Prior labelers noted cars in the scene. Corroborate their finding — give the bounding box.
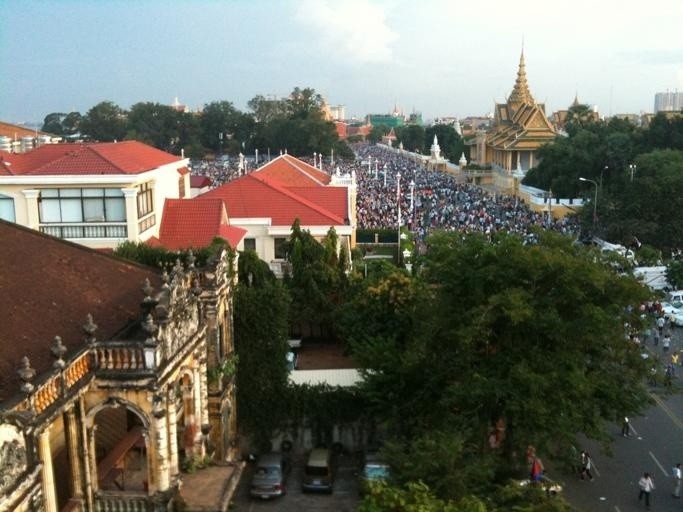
[660,290,683,327]
[354,455,394,495]
[249,453,292,501]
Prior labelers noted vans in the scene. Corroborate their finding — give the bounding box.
[302,447,337,494]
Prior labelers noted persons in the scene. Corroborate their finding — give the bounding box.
[191,156,268,189]
[671,463,682,498]
[637,472,655,506]
[623,299,680,387]
[297,141,578,244]
[621,417,633,438]
[580,454,594,482]
[580,450,585,465]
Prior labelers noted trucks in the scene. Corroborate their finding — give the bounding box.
[621,266,674,294]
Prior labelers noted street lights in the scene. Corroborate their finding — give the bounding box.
[629,163,636,181]
[312,149,334,170]
[578,177,598,227]
[180,148,185,159]
[600,166,609,187]
[548,187,553,225]
[367,155,415,210]
[238,147,271,177]
